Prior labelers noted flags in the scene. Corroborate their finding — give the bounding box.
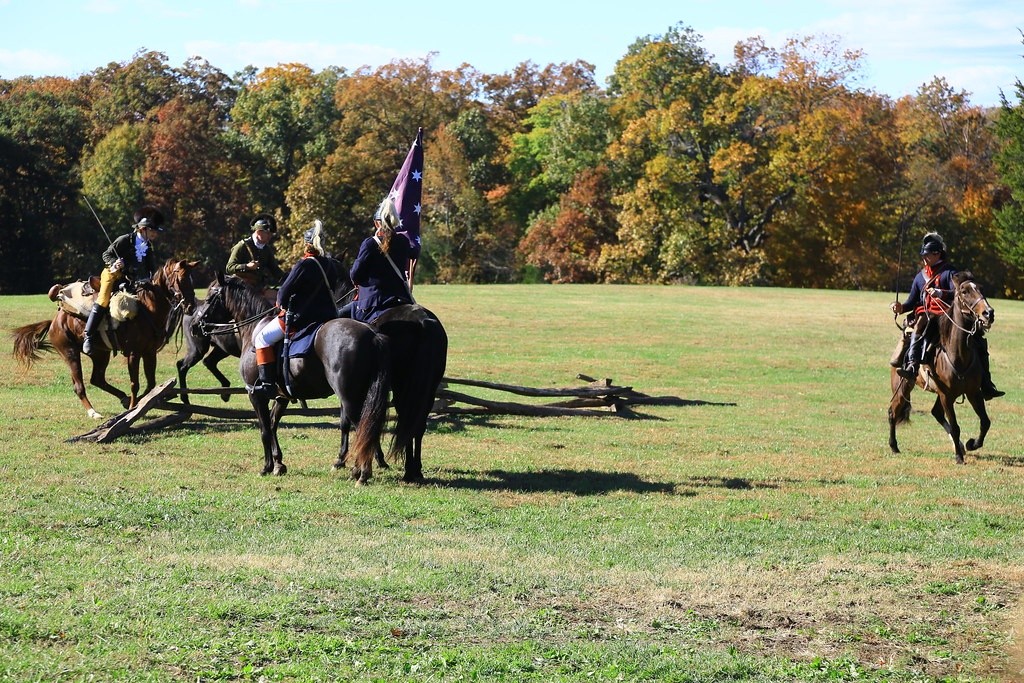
[386,131,424,292]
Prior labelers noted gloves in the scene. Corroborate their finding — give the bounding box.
[927,288,942,298]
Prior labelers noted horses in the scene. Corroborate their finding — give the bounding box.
[887,270,995,466]
[10,259,203,420]
[177,248,449,488]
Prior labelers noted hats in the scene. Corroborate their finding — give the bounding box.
[373,195,403,231]
[305,219,326,257]
[250,213,277,233]
[920,232,946,256]
[131,205,169,234]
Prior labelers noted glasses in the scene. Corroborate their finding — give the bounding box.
[148,226,157,231]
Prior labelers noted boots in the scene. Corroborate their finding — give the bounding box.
[980,338,1005,401]
[245,361,277,399]
[82,302,107,356]
[896,334,923,383]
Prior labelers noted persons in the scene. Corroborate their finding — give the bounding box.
[245,198,418,395]
[83,207,289,356]
[893,233,1005,401]
